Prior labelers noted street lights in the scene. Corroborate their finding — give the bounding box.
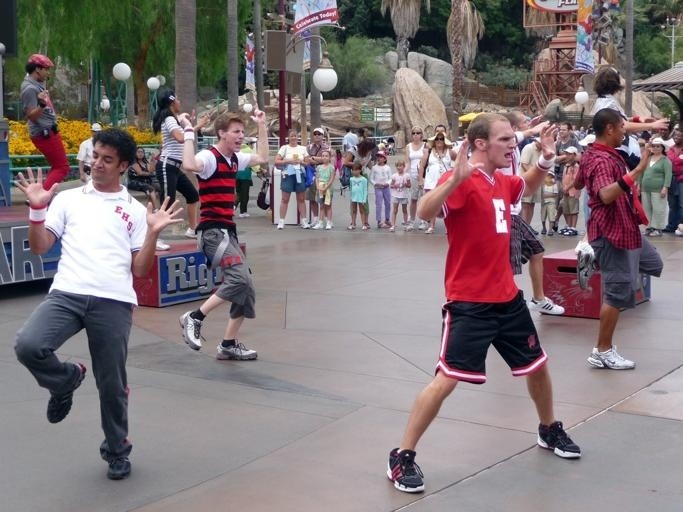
[658,16,682,67]
[237,94,252,112]
[206,96,225,116]
[278,21,346,148]
[98,61,129,128]
[573,72,594,104]
[147,74,166,128]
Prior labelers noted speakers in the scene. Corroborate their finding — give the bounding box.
[264,29,287,71]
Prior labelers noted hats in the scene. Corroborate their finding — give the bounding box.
[89,122,103,132]
[383,140,387,143]
[637,138,646,143]
[426,132,454,149]
[28,54,54,67]
[578,134,596,147]
[313,128,324,135]
[375,150,387,164]
[645,138,669,153]
[564,145,577,155]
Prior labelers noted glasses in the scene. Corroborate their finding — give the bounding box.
[434,137,444,141]
[412,132,421,135]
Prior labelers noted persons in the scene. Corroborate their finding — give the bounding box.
[152,88,211,249]
[19,54,72,191]
[126,147,162,211]
[515,113,683,237]
[77,123,103,184]
[385,112,580,494]
[494,121,564,315]
[573,109,664,370]
[233,125,471,234]
[11,128,184,481]
[178,108,269,361]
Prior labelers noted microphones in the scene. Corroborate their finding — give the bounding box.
[46,76,50,81]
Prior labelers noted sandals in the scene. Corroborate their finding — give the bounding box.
[559,226,577,236]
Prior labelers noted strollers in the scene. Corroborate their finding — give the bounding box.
[384,136,395,155]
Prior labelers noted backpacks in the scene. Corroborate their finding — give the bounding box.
[305,165,316,188]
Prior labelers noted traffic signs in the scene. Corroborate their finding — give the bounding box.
[360,105,392,123]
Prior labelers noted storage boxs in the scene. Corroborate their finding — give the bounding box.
[540,238,652,323]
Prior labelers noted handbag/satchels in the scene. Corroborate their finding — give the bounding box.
[417,160,428,178]
[339,164,351,187]
[257,190,270,210]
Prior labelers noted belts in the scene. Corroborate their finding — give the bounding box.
[160,157,181,169]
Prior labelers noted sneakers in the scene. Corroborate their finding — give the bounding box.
[155,238,170,251]
[642,228,663,237]
[362,223,371,229]
[347,223,356,230]
[386,448,424,493]
[301,218,335,230]
[530,224,559,236]
[529,297,564,315]
[585,344,635,369]
[575,241,601,290]
[107,455,131,480]
[238,213,250,218]
[215,340,257,360]
[377,220,436,234]
[185,227,198,239]
[178,309,208,351]
[536,420,581,459]
[47,362,87,424]
[277,218,285,229]
